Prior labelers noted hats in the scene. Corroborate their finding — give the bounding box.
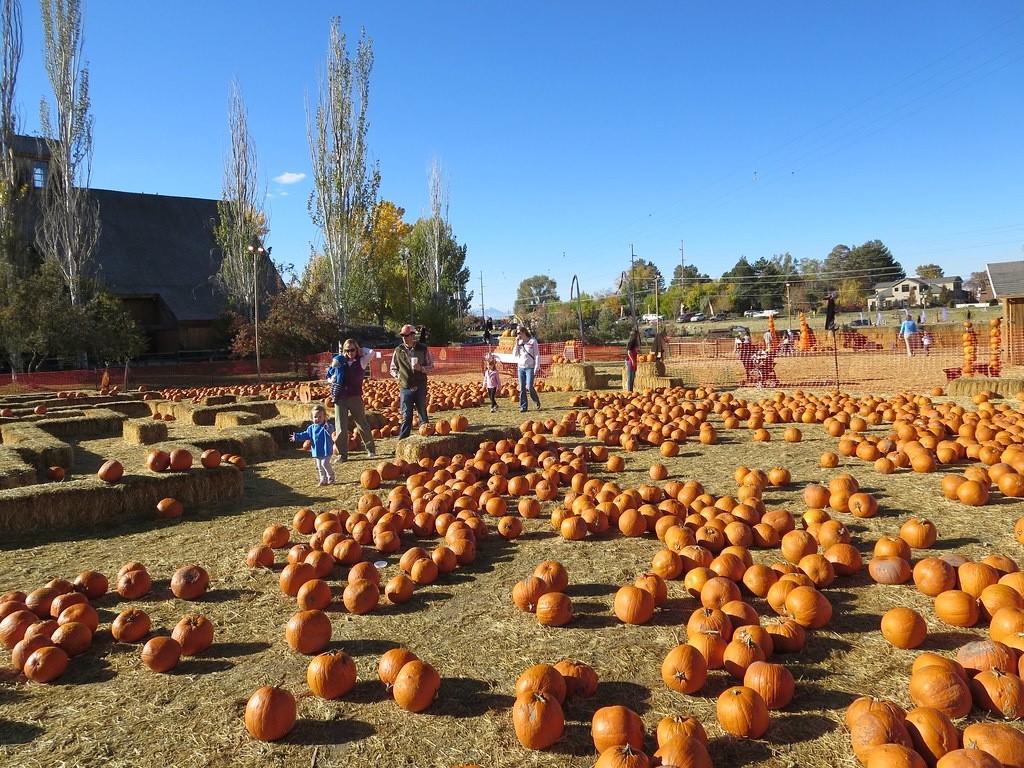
[399,324,416,336]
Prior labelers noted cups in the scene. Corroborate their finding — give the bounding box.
[411,357,418,369]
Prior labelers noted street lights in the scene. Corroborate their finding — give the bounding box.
[398,247,413,325]
[247,245,264,375]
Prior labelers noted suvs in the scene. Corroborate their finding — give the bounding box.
[642,314,666,322]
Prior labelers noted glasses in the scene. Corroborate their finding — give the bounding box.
[345,347,356,352]
[516,332,524,335]
[401,333,414,337]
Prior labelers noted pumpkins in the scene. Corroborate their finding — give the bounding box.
[565,340,576,347]
[502,328,517,337]
[0,354,1024,768]
[962,317,1001,380]
[769,312,810,352]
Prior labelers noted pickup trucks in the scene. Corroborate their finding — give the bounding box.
[459,336,500,346]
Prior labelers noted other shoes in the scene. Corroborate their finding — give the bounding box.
[390,449,396,455]
[535,401,540,409]
[520,408,527,412]
[327,473,334,484]
[490,404,498,413]
[367,452,374,460]
[319,477,327,486]
[335,456,347,463]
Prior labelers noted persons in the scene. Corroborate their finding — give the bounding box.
[625,330,640,391]
[513,326,540,412]
[921,331,931,356]
[736,333,750,348]
[288,404,334,487]
[389,323,434,454]
[483,359,501,413]
[782,328,793,349]
[898,315,917,357]
[763,329,773,349]
[326,338,375,462]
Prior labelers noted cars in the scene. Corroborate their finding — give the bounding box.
[709,313,726,322]
[689,312,707,322]
[676,312,696,322]
[744,310,757,318]
